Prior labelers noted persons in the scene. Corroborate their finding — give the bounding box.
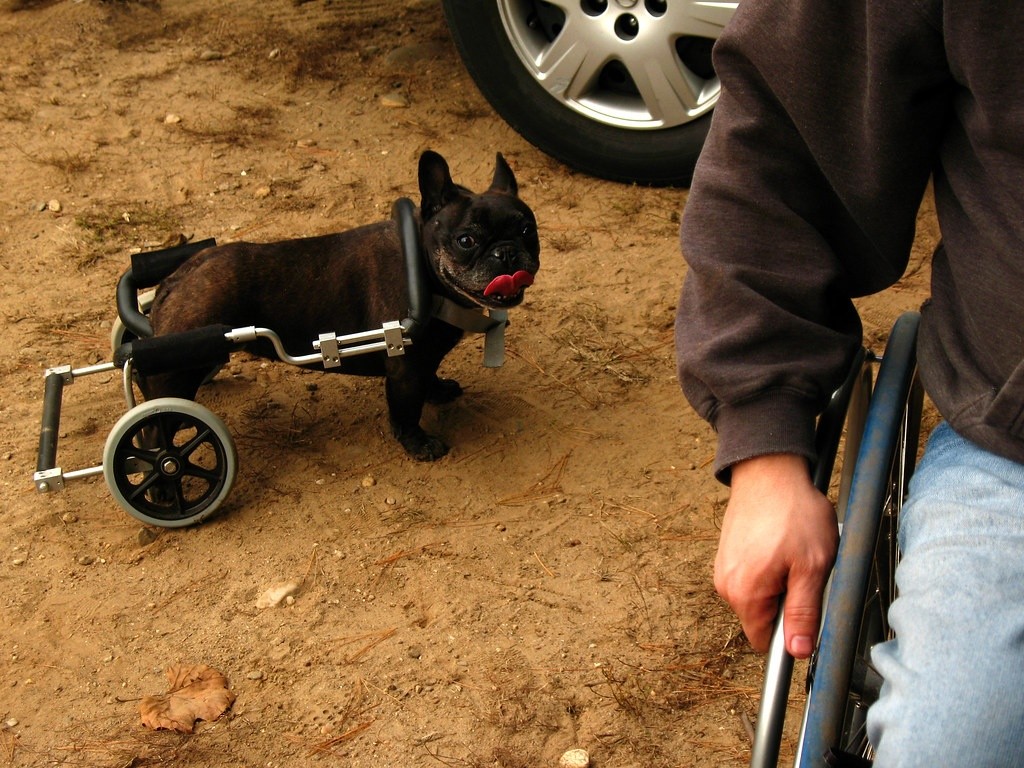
[675,1,1024,767]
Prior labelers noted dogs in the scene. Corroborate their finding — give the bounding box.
[133,149,541,502]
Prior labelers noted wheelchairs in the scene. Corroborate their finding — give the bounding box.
[746,310,925,768]
